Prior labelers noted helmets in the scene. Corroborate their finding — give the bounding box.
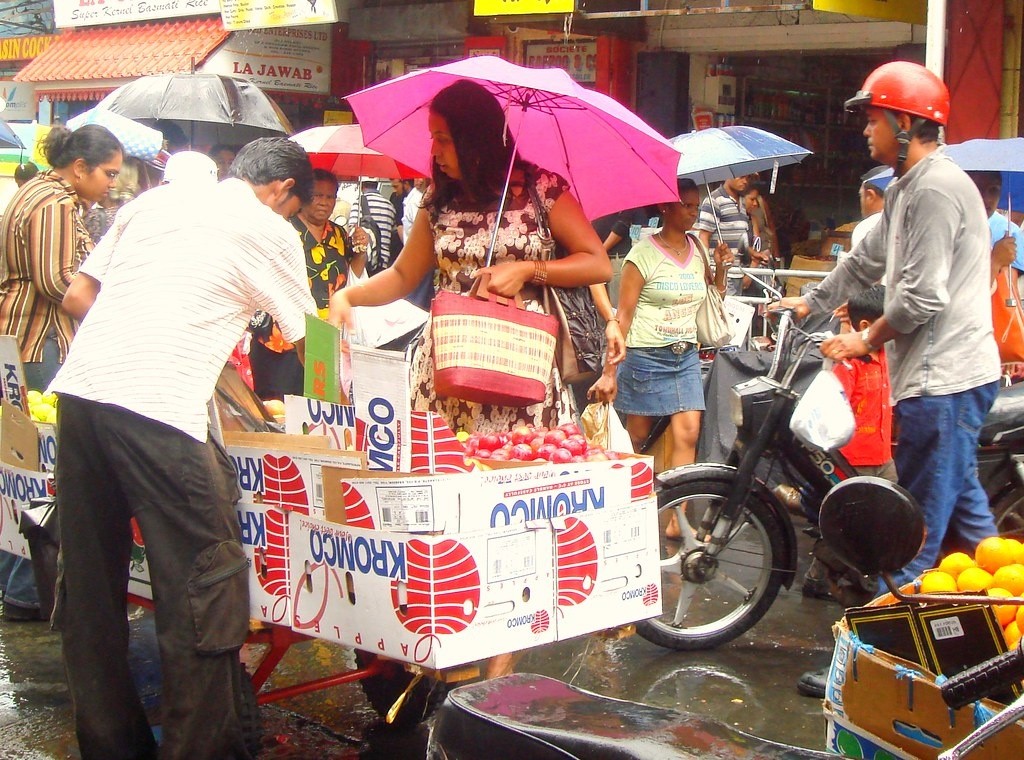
[844,60,949,128]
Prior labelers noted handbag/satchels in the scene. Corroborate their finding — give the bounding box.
[581,399,633,454]
[429,269,560,408]
[530,185,605,384]
[688,232,732,347]
[788,330,855,452]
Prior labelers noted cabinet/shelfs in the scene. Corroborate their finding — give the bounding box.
[738,75,882,189]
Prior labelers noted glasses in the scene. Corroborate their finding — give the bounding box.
[94,164,119,180]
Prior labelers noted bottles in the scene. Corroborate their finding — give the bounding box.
[706,52,735,76]
[747,130,873,187]
[743,52,874,87]
[715,112,736,128]
[747,87,870,128]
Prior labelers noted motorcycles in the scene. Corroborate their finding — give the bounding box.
[428,478,1024,760]
[634,289,1023,653]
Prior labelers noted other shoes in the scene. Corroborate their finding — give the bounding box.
[802,571,862,608]
[798,663,833,696]
[772,482,808,517]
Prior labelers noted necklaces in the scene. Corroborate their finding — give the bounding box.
[658,232,687,256]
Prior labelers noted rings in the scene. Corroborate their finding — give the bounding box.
[363,236,365,239]
[832,350,839,354]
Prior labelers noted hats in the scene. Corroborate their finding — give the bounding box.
[165,152,218,187]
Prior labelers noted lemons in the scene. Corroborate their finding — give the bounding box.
[25,388,59,423]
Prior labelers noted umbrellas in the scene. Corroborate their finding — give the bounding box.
[0,117,27,149]
[95,59,294,154]
[866,136,1024,309]
[286,124,430,250]
[343,54,683,271]
[663,125,812,269]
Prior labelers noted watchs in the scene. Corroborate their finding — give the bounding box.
[862,327,880,351]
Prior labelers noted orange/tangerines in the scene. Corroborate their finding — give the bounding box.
[918,536,1024,652]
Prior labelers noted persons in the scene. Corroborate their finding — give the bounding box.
[47,136,317,759]
[760,58,1024,693]
[772,167,1024,601]
[0,80,777,683]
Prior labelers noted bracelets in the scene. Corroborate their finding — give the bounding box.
[530,260,548,287]
[606,318,620,324]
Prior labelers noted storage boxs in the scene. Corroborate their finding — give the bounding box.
[786,221,861,297]
[1,403,661,670]
[824,584,1024,760]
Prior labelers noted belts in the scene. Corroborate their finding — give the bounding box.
[666,343,690,354]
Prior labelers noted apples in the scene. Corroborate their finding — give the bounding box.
[263,398,285,422]
[462,423,619,464]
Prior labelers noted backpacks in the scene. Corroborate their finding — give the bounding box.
[348,196,382,271]
